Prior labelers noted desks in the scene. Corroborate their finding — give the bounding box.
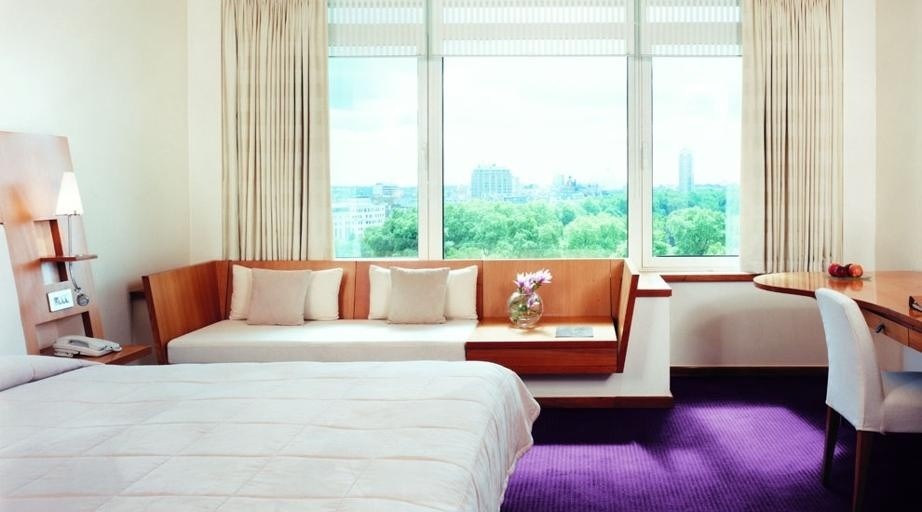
[752,268,922,428]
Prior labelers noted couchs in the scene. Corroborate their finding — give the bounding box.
[141,258,640,376]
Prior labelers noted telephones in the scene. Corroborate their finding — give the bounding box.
[53,335,121,357]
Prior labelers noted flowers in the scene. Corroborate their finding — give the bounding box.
[510,264,553,321]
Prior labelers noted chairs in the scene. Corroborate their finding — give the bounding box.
[815,284,922,511]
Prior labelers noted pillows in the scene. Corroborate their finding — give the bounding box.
[368,262,391,321]
[388,266,452,325]
[445,264,479,322]
[304,267,345,322]
[246,268,315,325]
[230,262,252,322]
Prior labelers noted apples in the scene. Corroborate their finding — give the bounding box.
[828,263,862,277]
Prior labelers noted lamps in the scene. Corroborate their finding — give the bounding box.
[54,171,91,306]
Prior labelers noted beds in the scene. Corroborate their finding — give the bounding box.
[1,361,519,511]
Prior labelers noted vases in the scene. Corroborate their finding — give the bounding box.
[507,291,544,330]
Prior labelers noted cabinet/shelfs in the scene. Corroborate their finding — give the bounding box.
[0,130,152,364]
